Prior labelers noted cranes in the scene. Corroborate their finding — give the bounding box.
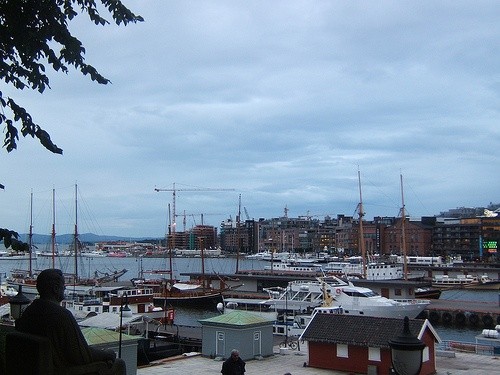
[295,209,337,221]
[153,182,237,233]
[175,208,226,234]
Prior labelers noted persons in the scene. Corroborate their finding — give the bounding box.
[15,269,127,375]
[221,349,246,375]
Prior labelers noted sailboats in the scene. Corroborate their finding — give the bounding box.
[10,184,128,285]
[128,204,245,306]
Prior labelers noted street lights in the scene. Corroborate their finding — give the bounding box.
[164,281,172,333]
[118,292,132,358]
[10,283,30,323]
[385,316,425,375]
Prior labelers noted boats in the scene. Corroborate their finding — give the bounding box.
[264,273,431,320]
[0,248,128,261]
[279,336,297,350]
[246,250,327,263]
[216,295,355,337]
[431,274,472,288]
[394,255,458,272]
[61,286,175,327]
[324,260,404,281]
[140,268,173,276]
[1,270,30,306]
[264,263,323,274]
